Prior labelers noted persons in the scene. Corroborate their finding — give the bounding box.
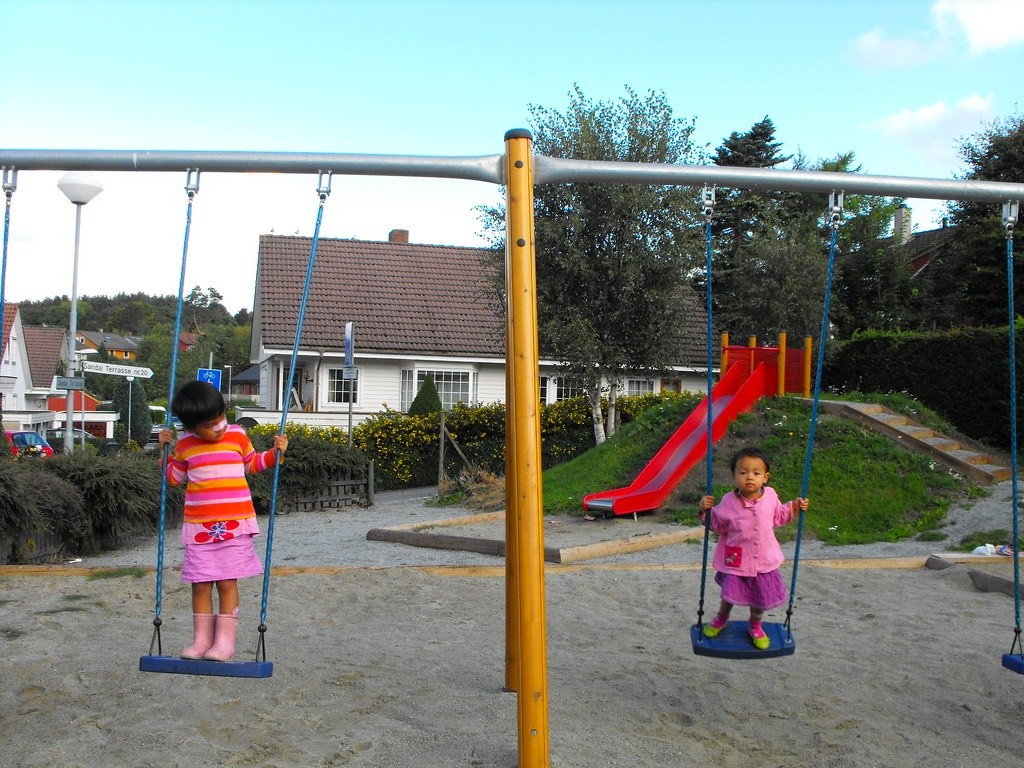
[159,381,288,661]
[699,447,809,650]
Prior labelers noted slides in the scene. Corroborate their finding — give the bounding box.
[582,359,767,515]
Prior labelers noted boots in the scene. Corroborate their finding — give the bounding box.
[204,614,237,660]
[180,614,217,659]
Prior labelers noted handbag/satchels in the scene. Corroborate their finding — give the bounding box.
[972,544,996,556]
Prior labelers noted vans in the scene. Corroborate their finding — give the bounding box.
[146,405,169,442]
[38,428,124,456]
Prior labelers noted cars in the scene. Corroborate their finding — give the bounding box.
[2,430,54,462]
[171,416,183,430]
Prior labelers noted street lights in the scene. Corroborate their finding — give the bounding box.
[56,170,103,456]
[223,365,232,410]
[126,377,136,447]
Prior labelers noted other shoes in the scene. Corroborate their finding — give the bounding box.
[748,625,769,649]
[703,618,727,637]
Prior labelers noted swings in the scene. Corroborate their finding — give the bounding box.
[1000,223,1024,673]
[690,207,840,659]
[138,189,329,679]
